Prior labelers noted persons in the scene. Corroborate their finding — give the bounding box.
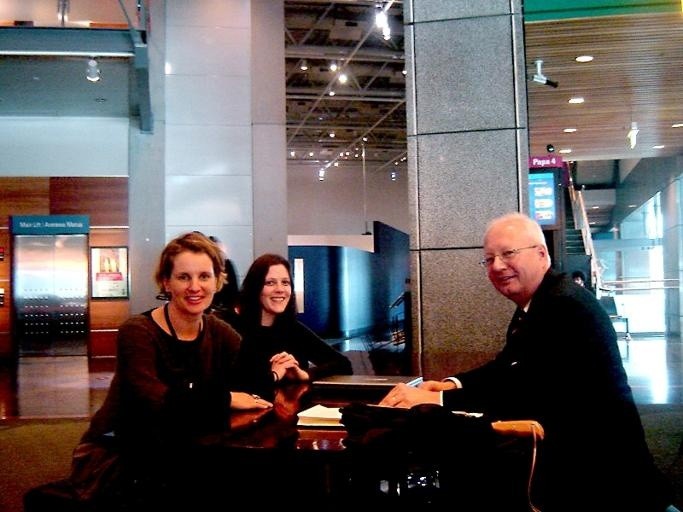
[215,254,354,395]
[71,232,273,511]
[572,270,596,295]
[376,212,656,512]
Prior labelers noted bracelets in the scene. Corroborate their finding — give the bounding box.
[271,371,279,383]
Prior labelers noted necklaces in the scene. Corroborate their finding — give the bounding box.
[163,303,202,390]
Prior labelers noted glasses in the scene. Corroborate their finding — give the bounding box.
[478,244,539,266]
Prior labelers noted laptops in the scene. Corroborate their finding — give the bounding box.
[311,374,424,397]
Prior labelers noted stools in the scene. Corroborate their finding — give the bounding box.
[21,479,89,511]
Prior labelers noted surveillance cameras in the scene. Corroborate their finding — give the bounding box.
[531,75,559,88]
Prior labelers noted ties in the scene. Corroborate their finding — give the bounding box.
[509,306,527,331]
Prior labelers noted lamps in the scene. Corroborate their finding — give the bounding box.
[86,56,100,82]
[527,61,559,89]
[287,0,407,182]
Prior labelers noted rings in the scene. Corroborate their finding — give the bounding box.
[253,394,260,399]
